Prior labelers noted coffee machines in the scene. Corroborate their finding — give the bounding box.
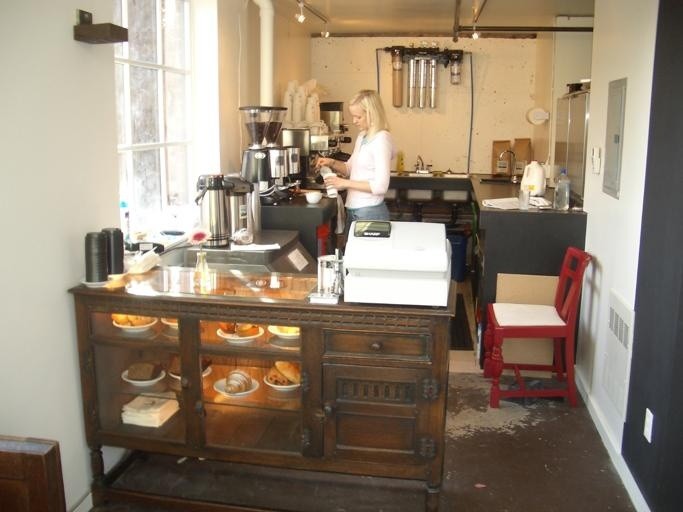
[236,105,302,206]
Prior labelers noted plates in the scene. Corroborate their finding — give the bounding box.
[109,269,127,280]
[160,317,180,331]
[79,277,112,287]
[166,363,211,381]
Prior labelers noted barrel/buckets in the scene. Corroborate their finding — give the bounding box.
[519,159,548,196]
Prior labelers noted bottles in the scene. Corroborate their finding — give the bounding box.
[195,251,213,296]
[551,167,570,211]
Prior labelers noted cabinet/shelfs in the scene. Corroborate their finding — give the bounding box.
[67,269,458,510]
[468,176,586,371]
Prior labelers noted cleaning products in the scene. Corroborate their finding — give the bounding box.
[396,150,405,173]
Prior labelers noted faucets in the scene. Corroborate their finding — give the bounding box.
[499,150,517,176]
[416,154,425,170]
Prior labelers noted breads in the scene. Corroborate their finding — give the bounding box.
[269,360,299,387]
[111,314,154,326]
[126,362,160,380]
[169,354,213,375]
[217,321,259,336]
[225,369,251,395]
[278,327,300,334]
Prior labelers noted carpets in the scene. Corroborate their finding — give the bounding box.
[449,293,474,350]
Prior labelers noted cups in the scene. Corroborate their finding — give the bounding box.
[84,232,107,279]
[516,190,531,210]
[322,172,341,199]
[100,227,124,275]
[282,78,320,123]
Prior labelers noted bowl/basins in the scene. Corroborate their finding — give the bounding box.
[304,192,322,205]
[261,372,300,391]
[210,375,258,397]
[111,317,157,332]
[268,325,300,338]
[212,323,264,343]
[120,368,165,385]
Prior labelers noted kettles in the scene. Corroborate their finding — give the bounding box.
[193,174,229,248]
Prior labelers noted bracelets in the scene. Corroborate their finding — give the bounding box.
[330,159,336,168]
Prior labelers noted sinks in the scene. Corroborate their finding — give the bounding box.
[441,173,470,203]
[406,172,434,202]
[480,177,521,185]
[384,172,405,200]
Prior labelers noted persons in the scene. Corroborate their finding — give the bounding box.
[315,90,394,245]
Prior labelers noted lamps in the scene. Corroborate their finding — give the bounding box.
[294,1,330,41]
[470,21,480,42]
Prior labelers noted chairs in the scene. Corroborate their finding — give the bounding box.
[479,246,592,411]
